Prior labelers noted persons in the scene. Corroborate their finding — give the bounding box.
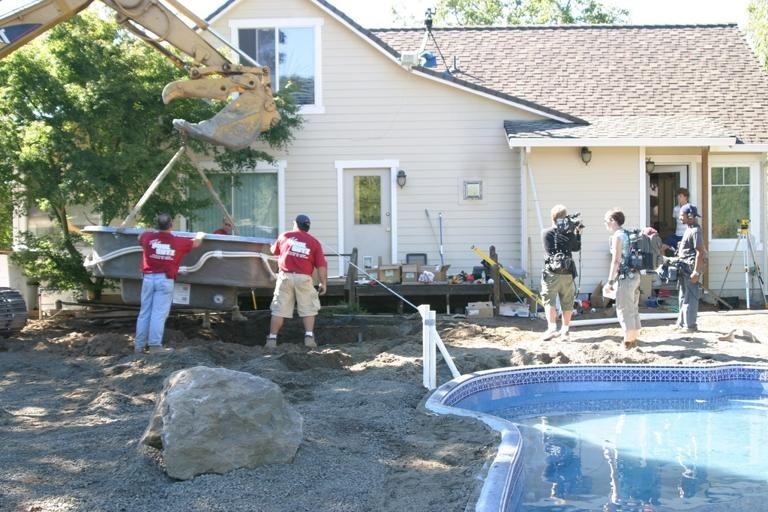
[604,208,641,350]
[540,204,581,340]
[663,188,689,246]
[202,216,247,330]
[264,214,327,350]
[667,204,704,334]
[134,214,205,356]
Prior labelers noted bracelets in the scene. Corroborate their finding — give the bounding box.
[607,279,616,285]
[693,271,701,277]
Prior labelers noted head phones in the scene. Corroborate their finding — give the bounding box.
[687,204,695,219]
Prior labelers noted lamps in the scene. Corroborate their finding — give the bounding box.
[646,157,654,174]
[397,170,407,189]
[581,147,591,165]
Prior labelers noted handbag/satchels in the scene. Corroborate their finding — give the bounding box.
[549,253,571,272]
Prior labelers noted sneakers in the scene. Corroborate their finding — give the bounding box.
[150,347,173,353]
[561,330,570,341]
[542,330,560,341]
[264,340,276,347]
[305,336,316,346]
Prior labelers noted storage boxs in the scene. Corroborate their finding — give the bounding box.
[466,301,496,319]
[363,254,451,284]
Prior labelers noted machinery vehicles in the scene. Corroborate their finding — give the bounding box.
[0,1,280,344]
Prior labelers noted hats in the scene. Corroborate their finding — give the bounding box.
[293,214,309,224]
[680,204,700,217]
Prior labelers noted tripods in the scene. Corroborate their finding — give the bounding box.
[714,219,768,309]
[418,7,452,72]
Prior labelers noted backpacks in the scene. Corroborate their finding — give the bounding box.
[620,227,662,270]
